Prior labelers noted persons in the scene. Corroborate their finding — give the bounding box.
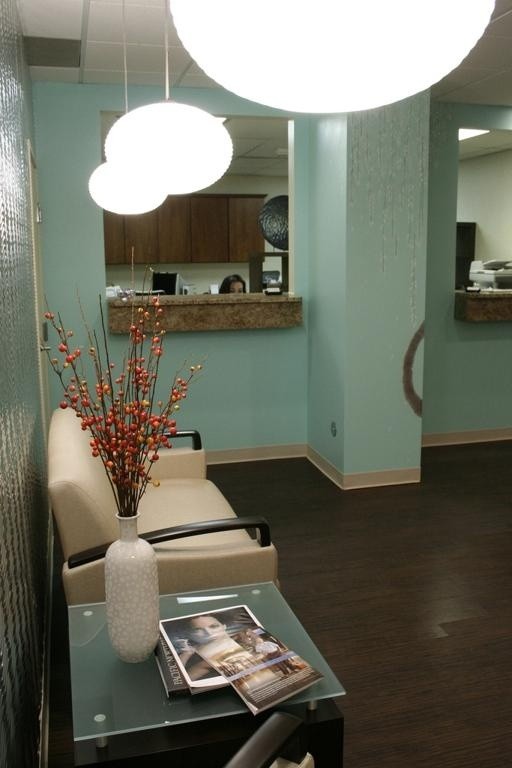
[254,635,301,677]
[217,274,247,293]
[188,615,229,643]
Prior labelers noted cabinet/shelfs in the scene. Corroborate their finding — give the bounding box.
[102,192,267,265]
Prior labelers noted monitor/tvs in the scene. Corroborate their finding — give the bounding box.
[152,272,179,295]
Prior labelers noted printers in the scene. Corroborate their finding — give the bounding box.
[467,259,512,292]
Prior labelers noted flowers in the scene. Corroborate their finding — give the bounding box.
[33,240,207,517]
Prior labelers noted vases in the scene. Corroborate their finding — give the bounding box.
[101,512,164,664]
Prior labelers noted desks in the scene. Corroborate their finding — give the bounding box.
[64,577,349,765]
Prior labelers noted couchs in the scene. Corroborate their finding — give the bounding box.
[44,399,285,605]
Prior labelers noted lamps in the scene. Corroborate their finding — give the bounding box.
[98,1,236,199]
[161,0,502,118]
[82,2,172,218]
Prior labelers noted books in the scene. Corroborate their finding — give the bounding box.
[158,605,266,696]
[151,635,190,700]
[189,622,326,717]
